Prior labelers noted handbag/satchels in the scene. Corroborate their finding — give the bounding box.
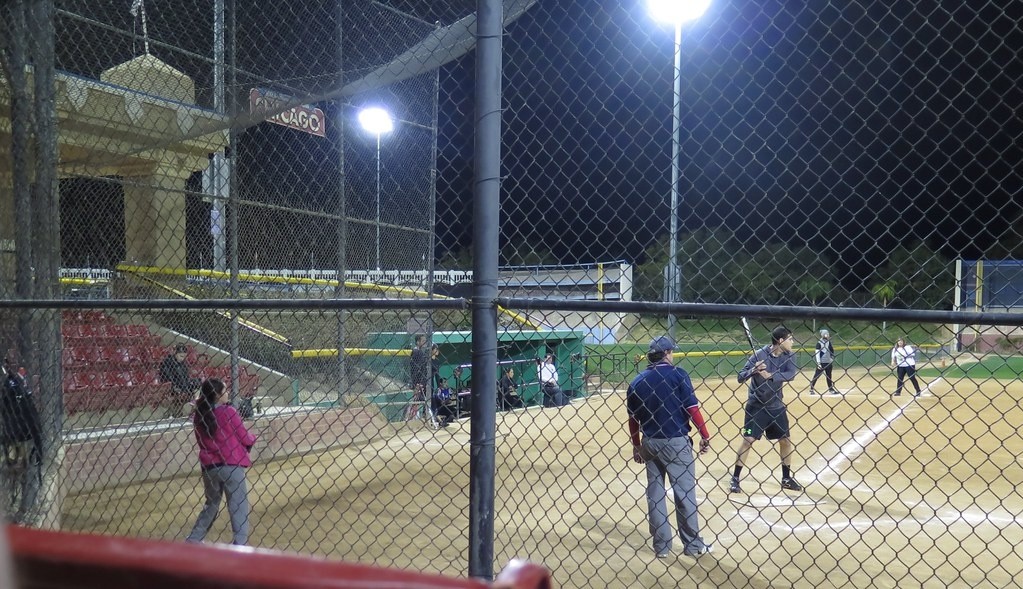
[0,374,42,443]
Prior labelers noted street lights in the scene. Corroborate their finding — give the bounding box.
[357,105,395,270]
[645,0,710,348]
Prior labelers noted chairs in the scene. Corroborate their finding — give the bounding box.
[0,308,260,414]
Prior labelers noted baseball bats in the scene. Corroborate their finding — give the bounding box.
[740,316,759,361]
[419,384,437,430]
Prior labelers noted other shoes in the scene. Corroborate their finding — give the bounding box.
[685,543,714,556]
[809,390,815,395]
[829,389,839,394]
[657,552,669,558]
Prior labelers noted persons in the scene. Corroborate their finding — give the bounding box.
[159,344,204,407]
[186,377,256,545]
[890,337,922,397]
[727,327,802,491]
[808,330,839,393]
[410,335,523,425]
[627,336,714,556]
[537,353,564,407]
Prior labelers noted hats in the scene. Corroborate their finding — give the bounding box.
[820,330,829,336]
[649,336,678,353]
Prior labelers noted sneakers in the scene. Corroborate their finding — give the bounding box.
[730,476,741,493]
[782,478,802,491]
